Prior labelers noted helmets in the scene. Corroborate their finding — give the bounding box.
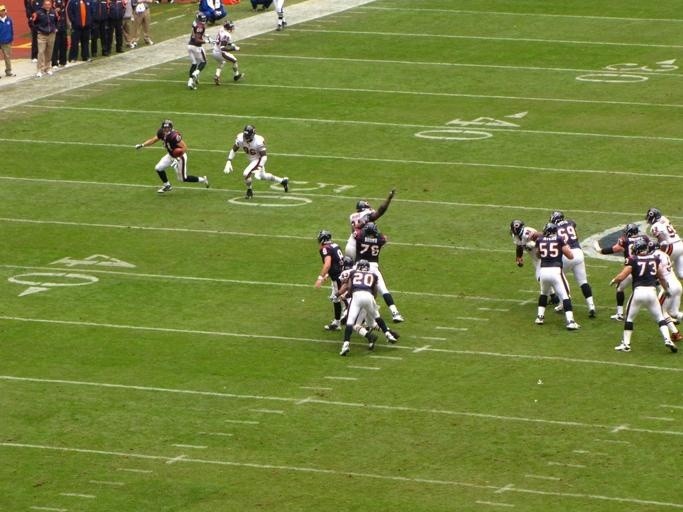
[511,219,524,235]
[223,20,234,32]
[624,223,655,255]
[318,229,332,243]
[196,13,206,24]
[542,222,557,237]
[341,255,371,271]
[160,120,174,134]
[242,123,257,139]
[550,210,564,224]
[355,200,378,236]
[645,207,661,224]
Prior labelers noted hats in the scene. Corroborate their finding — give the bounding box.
[0,3,6,12]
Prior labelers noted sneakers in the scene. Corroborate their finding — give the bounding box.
[146,39,155,45]
[534,298,582,330]
[664,319,683,354]
[127,41,138,49]
[245,188,254,200]
[324,323,352,356]
[156,184,172,194]
[587,306,624,322]
[213,72,245,86]
[187,68,200,91]
[280,176,289,193]
[613,343,632,352]
[202,175,209,188]
[36,47,126,78]
[276,21,287,31]
[367,313,405,350]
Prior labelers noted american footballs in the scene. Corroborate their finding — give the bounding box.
[173,148,184,157]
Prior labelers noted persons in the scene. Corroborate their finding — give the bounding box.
[508,217,559,308]
[210,20,245,86]
[546,211,597,318]
[592,205,683,352]
[198,1,226,26]
[186,11,210,90]
[132,119,209,194]
[524,222,581,330]
[314,187,405,357]
[222,123,290,200]
[272,0,287,32]
[0,0,152,79]
[249,0,273,11]
